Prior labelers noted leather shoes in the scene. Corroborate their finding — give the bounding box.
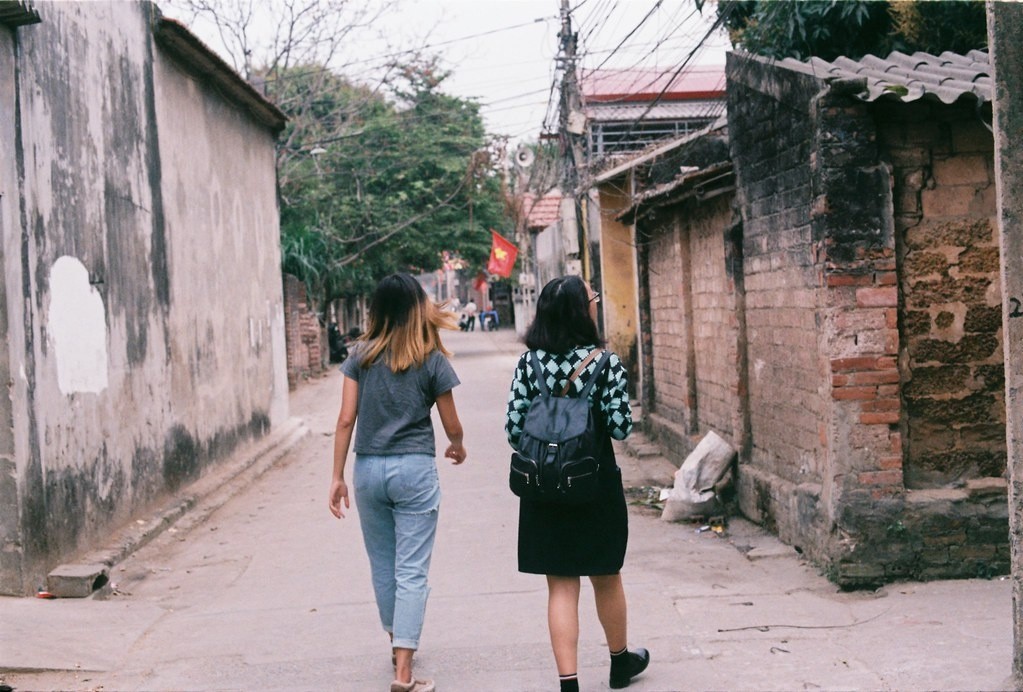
[609,648,650,689]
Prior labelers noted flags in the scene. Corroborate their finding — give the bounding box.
[487,233,517,278]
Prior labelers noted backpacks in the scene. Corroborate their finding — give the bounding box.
[508,349,612,507]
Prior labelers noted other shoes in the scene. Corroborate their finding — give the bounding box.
[391,675,436,692]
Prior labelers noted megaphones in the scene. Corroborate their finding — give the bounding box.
[516,147,534,167]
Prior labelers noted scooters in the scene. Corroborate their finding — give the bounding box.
[328,321,352,362]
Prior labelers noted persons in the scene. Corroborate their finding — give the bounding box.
[459,314,466,330]
[465,299,477,331]
[506,275,649,692]
[452,296,460,311]
[329,274,467,692]
[479,310,499,331]
